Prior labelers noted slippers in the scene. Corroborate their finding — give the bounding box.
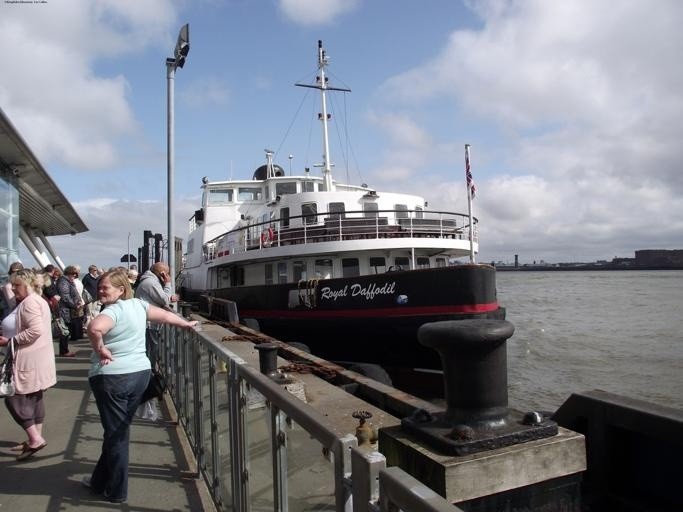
[12,441,48,460]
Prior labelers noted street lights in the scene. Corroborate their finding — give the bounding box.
[127,231,131,271]
[164,21,194,308]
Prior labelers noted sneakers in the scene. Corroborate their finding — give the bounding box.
[90,485,127,503]
[61,351,76,357]
[83,476,100,488]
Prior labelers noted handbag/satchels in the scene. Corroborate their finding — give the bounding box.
[0,357,16,397]
[139,371,168,403]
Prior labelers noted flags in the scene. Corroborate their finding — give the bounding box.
[464,150,476,201]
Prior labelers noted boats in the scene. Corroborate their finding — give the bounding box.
[176,36,503,399]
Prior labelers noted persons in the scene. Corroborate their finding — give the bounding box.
[0,262,139,357]
[134,262,179,421]
[0,269,57,462]
[82,269,199,503]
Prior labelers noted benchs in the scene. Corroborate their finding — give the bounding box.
[395,219,457,237]
[323,216,388,240]
